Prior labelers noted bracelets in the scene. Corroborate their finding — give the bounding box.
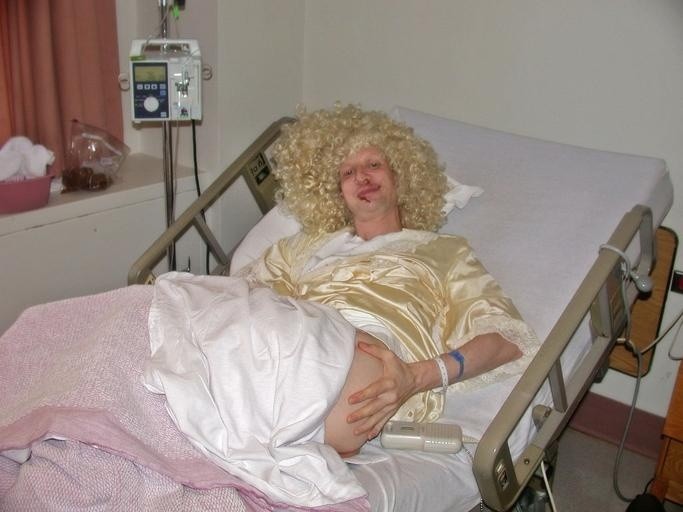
[446,349,466,383]
[430,353,448,396]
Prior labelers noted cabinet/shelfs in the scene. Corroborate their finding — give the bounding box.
[2,151,210,332]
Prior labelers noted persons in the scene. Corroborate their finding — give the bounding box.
[15,100,545,464]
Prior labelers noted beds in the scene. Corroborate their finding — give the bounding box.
[1,102,676,512]
